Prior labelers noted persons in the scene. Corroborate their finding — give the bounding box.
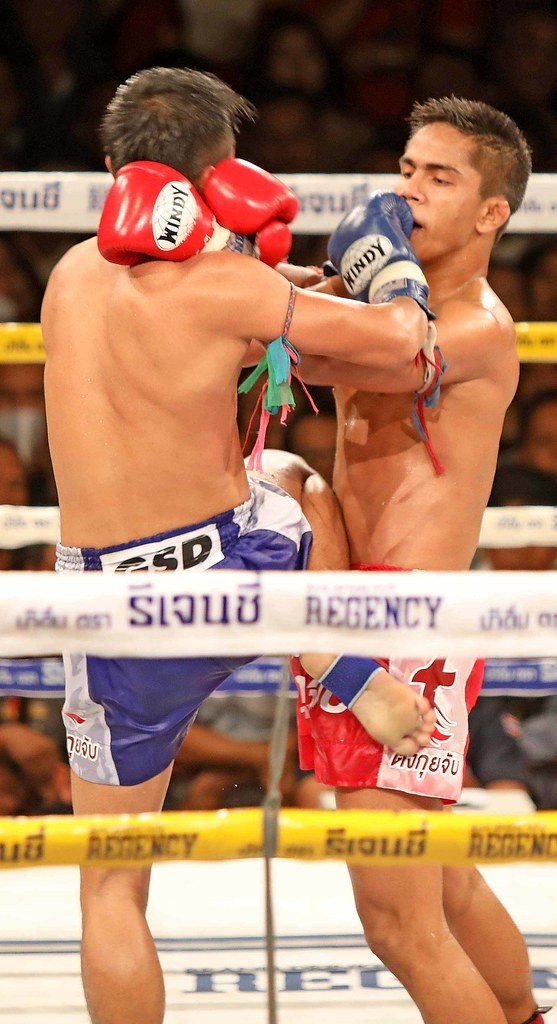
[98,98,553,1024]
[0,0,557,815]
[40,66,437,1024]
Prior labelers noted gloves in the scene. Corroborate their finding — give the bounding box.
[322,192,440,319]
[99,161,257,266]
[205,157,297,270]
